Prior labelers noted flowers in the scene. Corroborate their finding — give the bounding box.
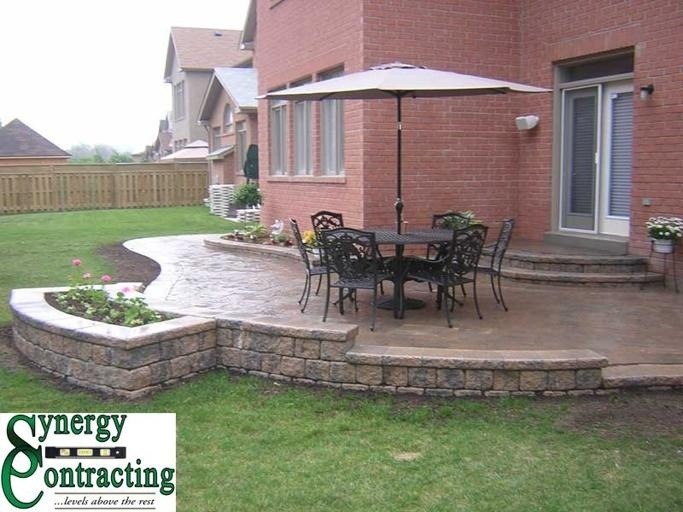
[644,216,682,240]
[53,259,164,327]
[289,211,512,332]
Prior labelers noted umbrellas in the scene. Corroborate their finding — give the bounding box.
[253,56,554,236]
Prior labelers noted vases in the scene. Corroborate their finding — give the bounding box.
[650,237,674,255]
